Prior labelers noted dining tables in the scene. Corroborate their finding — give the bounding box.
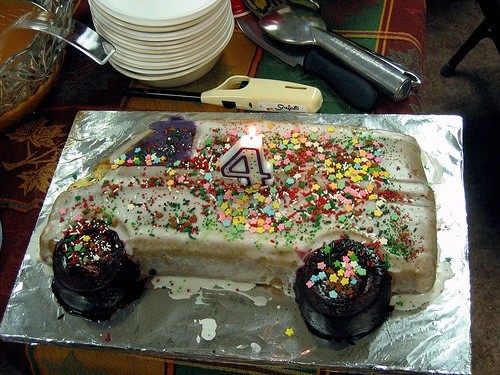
[0,0,426,375]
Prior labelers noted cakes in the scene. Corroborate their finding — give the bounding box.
[38,118,439,340]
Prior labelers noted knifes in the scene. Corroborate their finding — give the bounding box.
[235,17,380,113]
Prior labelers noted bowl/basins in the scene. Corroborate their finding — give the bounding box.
[0,0,74,131]
[85,0,235,89]
